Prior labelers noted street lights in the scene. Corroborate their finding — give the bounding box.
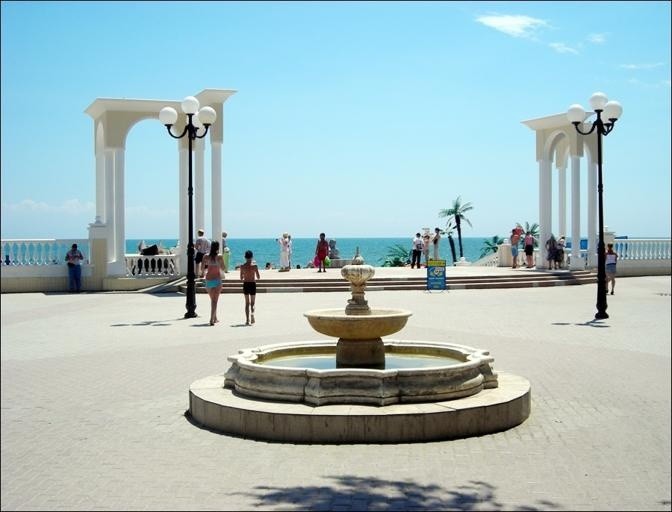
[567,91,625,320]
[158,95,218,317]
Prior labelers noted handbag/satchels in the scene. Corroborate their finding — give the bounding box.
[314,256,320,266]
[324,256,330,267]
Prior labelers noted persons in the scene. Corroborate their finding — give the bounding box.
[314,233,330,273]
[431,228,442,261]
[420,228,433,269]
[544,235,558,269]
[277,233,290,272]
[287,235,293,270]
[65,243,84,293]
[510,229,521,268]
[264,262,273,270]
[200,239,229,326]
[239,250,260,325]
[604,243,617,295]
[524,231,535,269]
[410,232,425,269]
[194,229,230,278]
[556,235,567,269]
